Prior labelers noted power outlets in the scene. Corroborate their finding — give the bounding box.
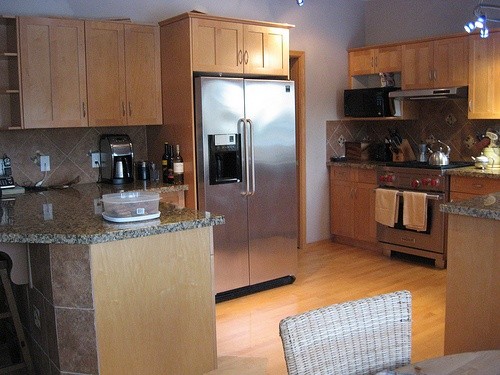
[40,156,50,172]
[92,153,100,168]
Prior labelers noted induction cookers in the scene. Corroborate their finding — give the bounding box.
[386,158,474,168]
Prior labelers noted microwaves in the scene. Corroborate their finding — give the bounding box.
[343,87,401,117]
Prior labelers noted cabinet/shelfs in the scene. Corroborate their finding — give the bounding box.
[330,166,378,252]
[0,11,296,130]
[450,176,500,203]
[372,172,449,270]
[347,28,500,121]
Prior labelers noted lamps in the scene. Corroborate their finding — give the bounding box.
[464,0,500,39]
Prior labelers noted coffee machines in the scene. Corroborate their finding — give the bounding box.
[99,133,134,185]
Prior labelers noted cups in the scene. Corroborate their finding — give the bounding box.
[138,160,150,180]
[484,147,500,170]
[149,162,159,181]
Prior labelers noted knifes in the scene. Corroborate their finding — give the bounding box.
[386,129,403,154]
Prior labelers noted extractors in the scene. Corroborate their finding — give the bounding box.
[388,84,468,100]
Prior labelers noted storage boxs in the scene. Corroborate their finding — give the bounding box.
[100,192,162,218]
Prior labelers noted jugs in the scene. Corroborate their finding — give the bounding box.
[471,151,495,170]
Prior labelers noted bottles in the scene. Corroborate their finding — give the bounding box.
[173,144,185,185]
[161,142,174,186]
[418,141,427,162]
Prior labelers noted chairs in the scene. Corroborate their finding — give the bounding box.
[279,290,411,375]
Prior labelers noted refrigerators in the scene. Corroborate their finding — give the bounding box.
[193,75,299,304]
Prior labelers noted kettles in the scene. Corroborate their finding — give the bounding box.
[427,143,450,166]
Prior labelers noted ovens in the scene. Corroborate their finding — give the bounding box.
[375,165,451,271]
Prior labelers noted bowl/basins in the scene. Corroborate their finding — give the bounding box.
[99,190,163,218]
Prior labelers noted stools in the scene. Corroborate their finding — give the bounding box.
[0,255,34,375]
